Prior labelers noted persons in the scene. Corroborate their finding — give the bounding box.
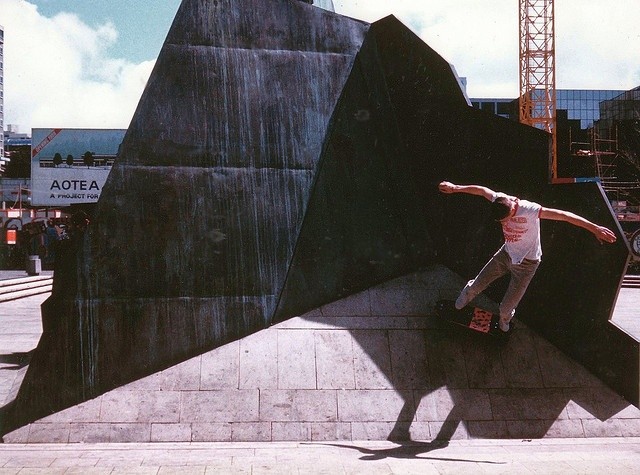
[438,181,617,332]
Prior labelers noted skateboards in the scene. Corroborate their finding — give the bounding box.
[435,299,515,344]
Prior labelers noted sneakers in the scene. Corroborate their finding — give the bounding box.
[499,316,509,332]
[455,284,470,310]
